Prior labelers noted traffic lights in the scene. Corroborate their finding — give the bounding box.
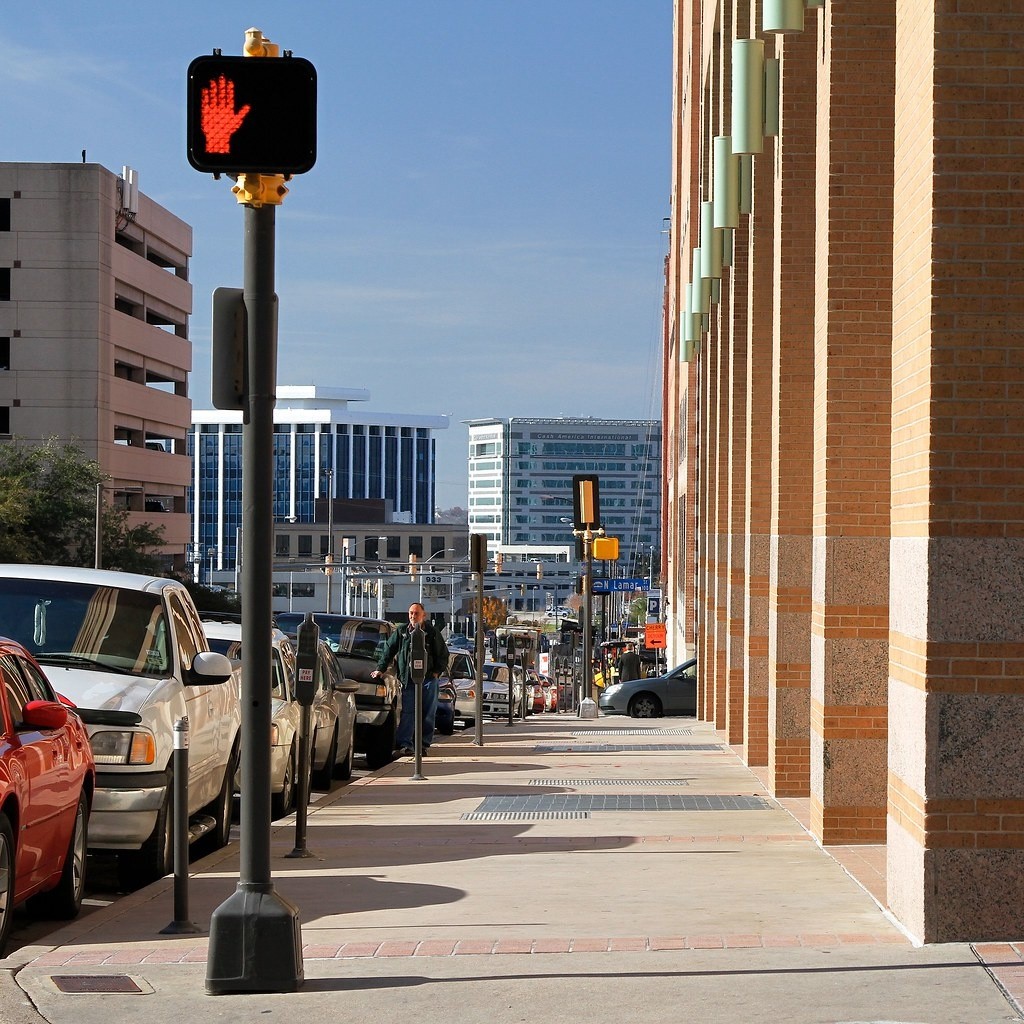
[185,52,314,176]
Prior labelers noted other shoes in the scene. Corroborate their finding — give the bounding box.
[422,748,428,756]
[391,750,402,758]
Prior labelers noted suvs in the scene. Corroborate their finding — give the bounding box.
[190,610,318,820]
[276,614,405,771]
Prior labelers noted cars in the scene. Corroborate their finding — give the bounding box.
[283,633,360,791]
[547,606,570,617]
[1,635,95,957]
[434,668,456,735]
[598,660,697,718]
[442,647,557,730]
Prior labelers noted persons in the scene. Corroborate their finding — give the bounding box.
[370,603,450,757]
[618,643,640,683]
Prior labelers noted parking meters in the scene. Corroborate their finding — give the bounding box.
[286,612,321,857]
[562,658,569,713]
[555,656,560,713]
[409,624,428,781]
[507,633,516,727]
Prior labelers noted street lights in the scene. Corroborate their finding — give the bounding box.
[539,493,607,662]
[340,536,387,614]
[532,586,538,625]
[96,483,143,570]
[420,549,455,606]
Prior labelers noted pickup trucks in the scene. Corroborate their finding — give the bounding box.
[0,564,242,896]
